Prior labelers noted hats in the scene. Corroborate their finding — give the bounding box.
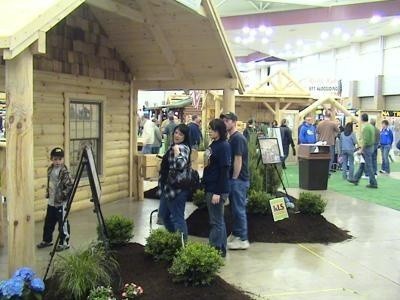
[51,148,63,156]
[220,112,237,121]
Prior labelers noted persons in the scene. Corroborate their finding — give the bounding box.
[156,124,192,248]
[37,147,74,250]
[203,118,231,261]
[219,112,250,250]
[137,108,393,188]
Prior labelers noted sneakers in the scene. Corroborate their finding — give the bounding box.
[227,238,250,250]
[56,244,70,252]
[36,240,53,249]
[227,234,240,242]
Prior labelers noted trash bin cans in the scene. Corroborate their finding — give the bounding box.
[297,143,332,190]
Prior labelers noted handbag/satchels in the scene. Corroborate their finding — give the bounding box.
[186,169,199,189]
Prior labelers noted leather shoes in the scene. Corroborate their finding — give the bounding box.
[346,179,358,185]
[365,185,377,188]
